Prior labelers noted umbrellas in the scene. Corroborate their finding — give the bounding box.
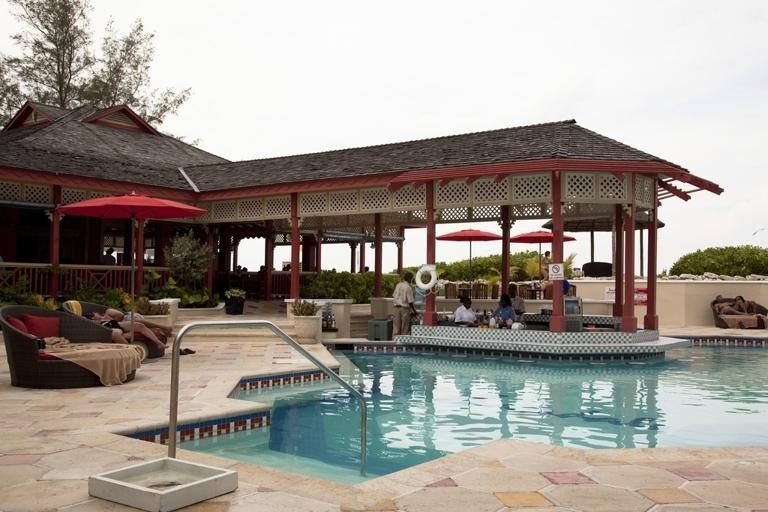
[55,190,208,347]
[435,226,504,280]
[510,230,577,282]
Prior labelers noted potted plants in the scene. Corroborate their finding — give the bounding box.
[289,295,323,344]
[224,288,247,315]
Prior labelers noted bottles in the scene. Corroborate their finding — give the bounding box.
[322,315,336,328]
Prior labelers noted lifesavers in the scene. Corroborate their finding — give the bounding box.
[415,266,437,290]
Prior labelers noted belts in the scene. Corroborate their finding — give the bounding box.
[395,305,402,307]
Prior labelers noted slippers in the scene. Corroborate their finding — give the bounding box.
[180,348,196,355]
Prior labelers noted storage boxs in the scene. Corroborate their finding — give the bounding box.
[367,317,393,340]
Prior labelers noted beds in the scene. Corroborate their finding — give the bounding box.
[711,298,768,329]
[0,300,171,388]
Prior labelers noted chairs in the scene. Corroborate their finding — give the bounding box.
[445,280,576,300]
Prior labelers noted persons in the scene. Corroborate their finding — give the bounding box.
[237,265,242,271]
[562,278,573,297]
[491,294,515,329]
[84,309,169,350]
[393,271,418,335]
[242,267,247,272]
[455,298,478,327]
[508,284,526,322]
[734,296,758,316]
[714,295,748,315]
[283,264,292,271]
[258,266,266,272]
[541,251,551,282]
[365,267,369,273]
[103,309,173,338]
